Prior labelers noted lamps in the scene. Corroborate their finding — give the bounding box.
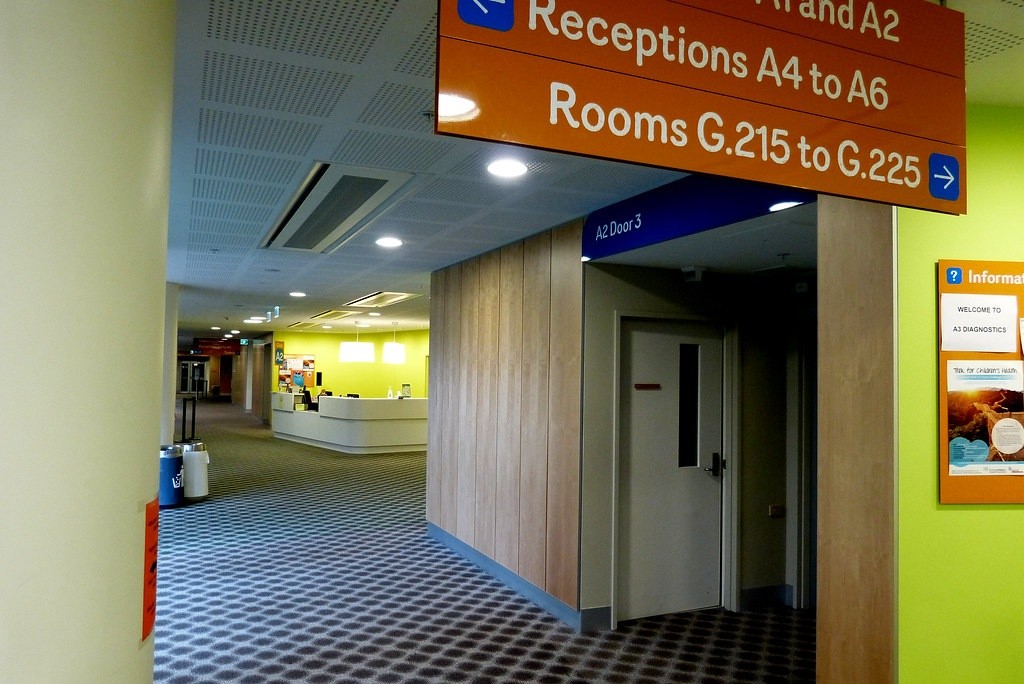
[339,321,374,362]
[382,322,406,363]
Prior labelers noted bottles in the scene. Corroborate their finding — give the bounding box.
[388,386,393,398]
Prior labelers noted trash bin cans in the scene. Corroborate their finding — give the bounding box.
[212,385,219,395]
[183,441,210,503]
[159,445,184,508]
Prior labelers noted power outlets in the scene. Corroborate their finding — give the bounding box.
[769,504,785,518]
